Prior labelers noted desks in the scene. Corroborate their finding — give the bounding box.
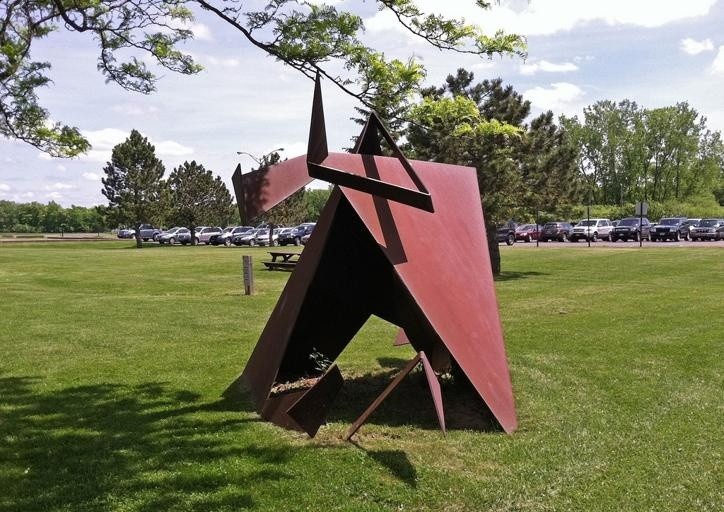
[266,251,302,271]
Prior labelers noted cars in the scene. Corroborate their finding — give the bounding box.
[496,218,724,245]
[117,223,316,247]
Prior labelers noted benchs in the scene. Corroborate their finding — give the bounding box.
[282,259,298,263]
[262,261,297,272]
